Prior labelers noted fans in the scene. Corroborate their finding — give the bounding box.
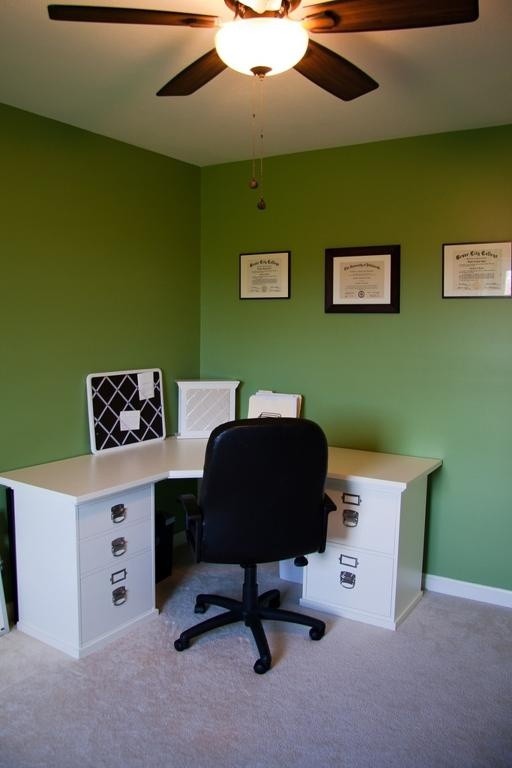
[46,0,483,101]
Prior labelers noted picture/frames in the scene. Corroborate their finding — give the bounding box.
[440,239,512,299]
[237,250,293,301]
[322,242,404,315]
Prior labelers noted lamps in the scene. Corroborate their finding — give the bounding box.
[211,2,309,81]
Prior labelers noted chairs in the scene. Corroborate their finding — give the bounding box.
[171,415,338,675]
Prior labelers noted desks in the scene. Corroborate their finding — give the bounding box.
[0,432,443,662]
